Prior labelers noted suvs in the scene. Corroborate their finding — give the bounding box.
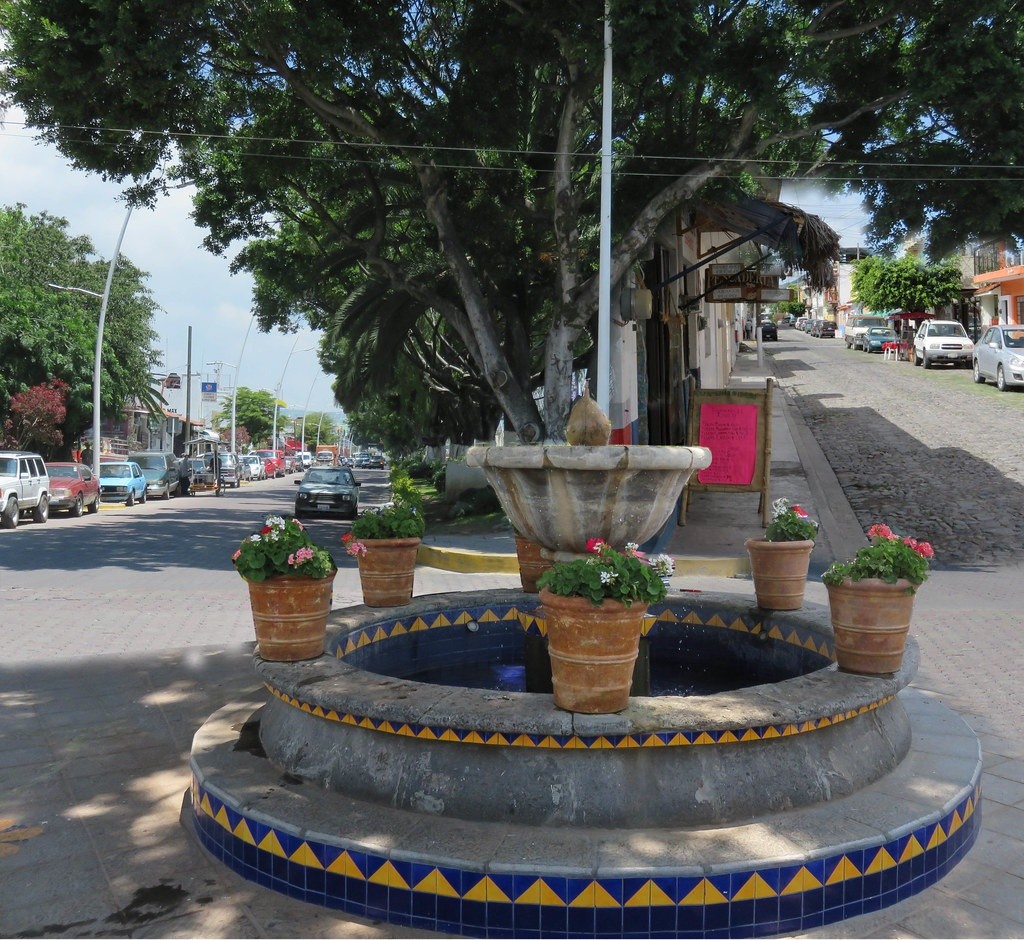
[126,450,181,501]
[913,318,974,369]
[0,450,52,529]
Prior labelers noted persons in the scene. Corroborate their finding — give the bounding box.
[81,442,93,467]
[735,319,740,343]
[211,451,222,495]
[745,319,753,339]
[179,455,192,496]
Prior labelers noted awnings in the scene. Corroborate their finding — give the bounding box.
[672,182,842,294]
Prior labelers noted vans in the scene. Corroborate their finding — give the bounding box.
[844,314,887,350]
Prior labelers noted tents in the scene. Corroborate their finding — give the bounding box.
[889,311,936,321]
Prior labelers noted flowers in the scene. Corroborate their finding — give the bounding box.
[539,543,675,603]
[764,498,819,544]
[229,516,348,582]
[820,523,935,583]
[343,498,429,555]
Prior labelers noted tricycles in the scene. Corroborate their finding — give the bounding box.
[187,468,226,498]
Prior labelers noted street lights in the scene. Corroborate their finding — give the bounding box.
[202,360,241,452]
[259,387,281,460]
[45,282,112,484]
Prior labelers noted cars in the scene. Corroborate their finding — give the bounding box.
[783,316,790,322]
[861,327,901,353]
[201,452,242,488]
[796,316,838,338]
[293,466,361,519]
[43,462,102,516]
[760,313,779,341]
[972,323,1024,391]
[789,318,796,326]
[88,462,148,507]
[186,459,209,471]
[237,443,388,480]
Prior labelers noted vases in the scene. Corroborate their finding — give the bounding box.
[358,537,420,609]
[546,593,648,714]
[830,571,919,675]
[248,573,337,664]
[744,532,813,610]
[519,535,557,594]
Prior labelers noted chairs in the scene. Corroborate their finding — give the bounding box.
[929,328,936,336]
